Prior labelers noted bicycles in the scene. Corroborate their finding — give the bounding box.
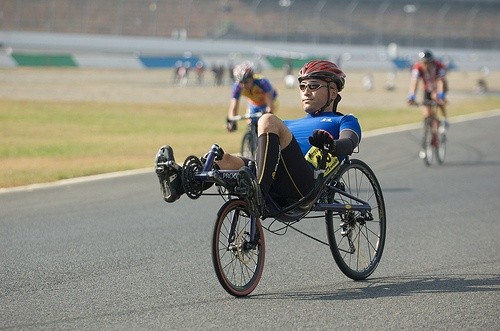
[231,112,262,157]
[155,144,386,297]
[418,99,446,166]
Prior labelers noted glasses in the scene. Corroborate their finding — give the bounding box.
[299,83,336,91]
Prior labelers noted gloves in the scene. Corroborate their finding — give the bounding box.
[227,121,237,132]
[309,129,333,150]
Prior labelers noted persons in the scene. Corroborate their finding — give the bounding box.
[226,62,278,150]
[156,59,361,220]
[171,57,490,96]
[409,50,450,159]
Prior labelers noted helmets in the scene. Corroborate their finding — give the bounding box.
[298,60,346,91]
[233,61,256,83]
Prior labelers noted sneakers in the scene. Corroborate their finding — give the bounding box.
[236,166,264,217]
[155,145,182,204]
